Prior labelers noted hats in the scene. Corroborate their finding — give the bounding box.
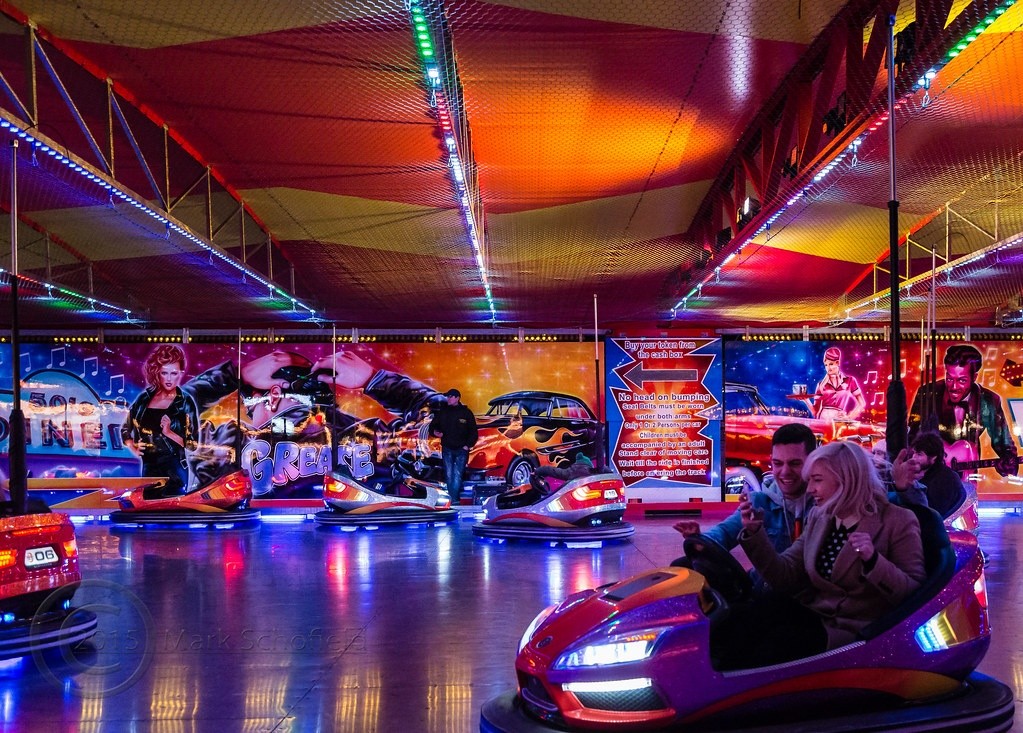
[872,439,887,455]
[442,388,461,398]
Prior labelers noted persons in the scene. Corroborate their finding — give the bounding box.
[670,423,928,566]
[872,439,887,468]
[532,452,594,482]
[428,389,478,504]
[912,433,960,516]
[719,440,924,670]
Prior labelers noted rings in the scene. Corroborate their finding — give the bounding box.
[856,547,860,552]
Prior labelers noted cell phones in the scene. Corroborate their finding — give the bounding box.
[742,482,755,522]
